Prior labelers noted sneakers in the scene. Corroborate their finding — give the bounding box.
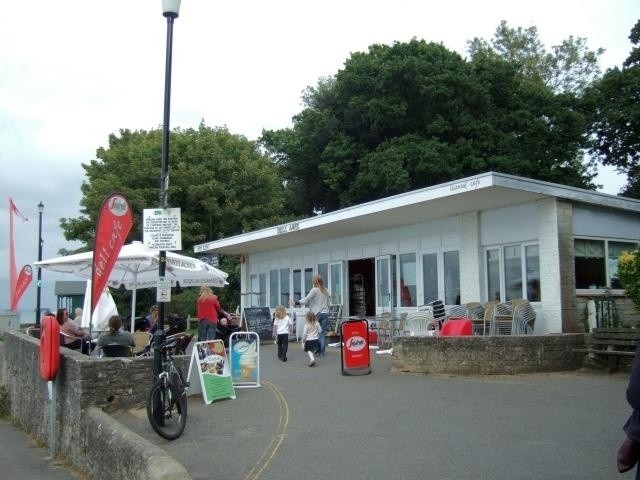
[278,354,287,361]
[309,361,316,367]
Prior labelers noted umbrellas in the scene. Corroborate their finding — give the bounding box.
[34,241,229,333]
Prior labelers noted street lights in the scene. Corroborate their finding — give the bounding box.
[152,0,181,427]
[35,200,45,328]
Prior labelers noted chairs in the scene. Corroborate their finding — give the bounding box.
[26,325,153,356]
[376,299,537,350]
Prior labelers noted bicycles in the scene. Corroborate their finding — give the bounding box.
[145,332,190,440]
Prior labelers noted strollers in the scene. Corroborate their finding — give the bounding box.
[215,313,242,349]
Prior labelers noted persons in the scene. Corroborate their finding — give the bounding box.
[97,315,136,349]
[74,307,83,326]
[145,305,159,325]
[295,274,331,357]
[56,309,96,355]
[272,305,292,361]
[617,346,640,480]
[220,317,229,328]
[198,286,220,340]
[301,311,322,367]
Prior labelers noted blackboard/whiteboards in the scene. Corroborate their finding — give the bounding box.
[324,304,342,334]
[243,307,276,343]
